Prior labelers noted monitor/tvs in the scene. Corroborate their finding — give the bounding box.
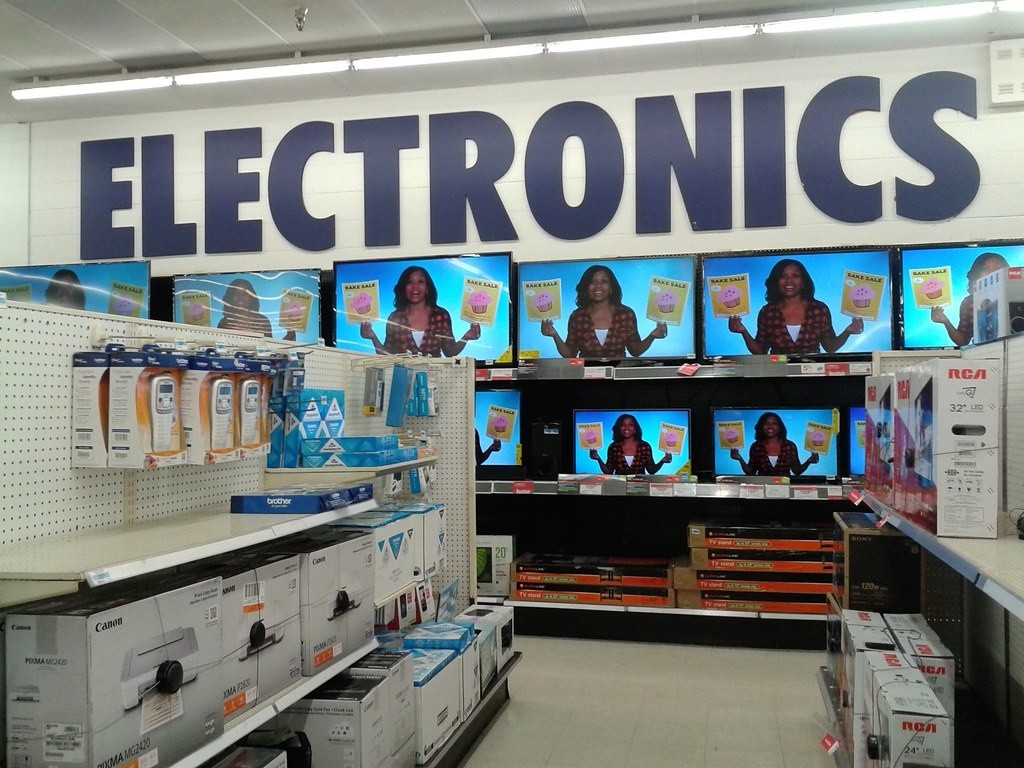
[698,245,897,361]
[474,389,526,470]
[333,251,516,369]
[573,409,691,481]
[896,238,1024,351]
[512,253,700,364]
[878,375,932,490]
[172,268,321,347]
[710,406,840,482]
[1,259,150,320]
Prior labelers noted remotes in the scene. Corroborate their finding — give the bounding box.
[240,378,261,447]
[150,376,175,452]
[208,379,234,450]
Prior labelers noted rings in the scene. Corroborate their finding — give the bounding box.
[669,460,672,462]
[860,328,862,331]
[546,328,548,331]
[663,333,666,336]
[498,448,500,449]
[476,334,479,337]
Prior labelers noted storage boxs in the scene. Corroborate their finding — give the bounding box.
[266,359,442,496]
[473,513,834,617]
[230,483,374,515]
[68,342,274,473]
[4,504,513,768]
[819,356,1002,768]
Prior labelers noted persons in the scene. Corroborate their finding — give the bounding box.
[589,414,672,475]
[44,270,85,311]
[728,259,864,355]
[931,252,1011,346]
[541,265,668,358]
[731,412,819,475]
[475,429,501,465]
[217,279,296,343]
[360,265,481,358]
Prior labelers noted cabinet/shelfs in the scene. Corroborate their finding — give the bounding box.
[815,332,1024,768]
[475,360,873,628]
[1,298,524,768]
